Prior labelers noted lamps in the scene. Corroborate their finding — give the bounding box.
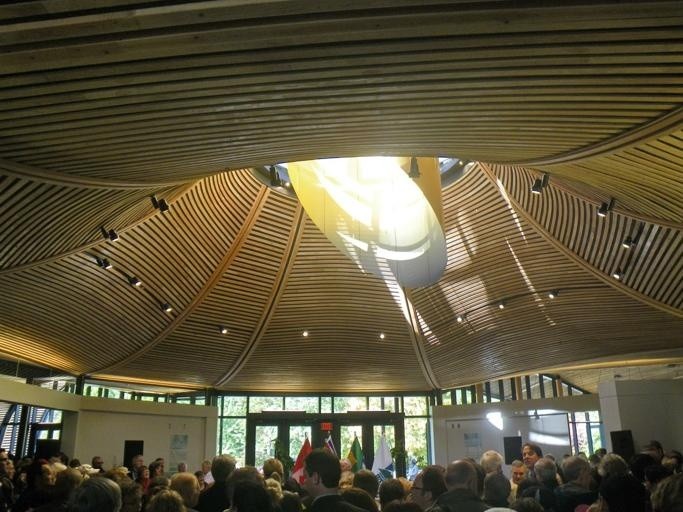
[147,191,170,213]
[546,289,560,300]
[128,276,173,312]
[408,156,421,180]
[218,324,227,334]
[93,225,119,270]
[597,198,636,249]
[529,173,551,194]
[612,267,622,279]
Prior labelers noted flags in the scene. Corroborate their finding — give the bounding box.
[327,435,337,455]
[370,434,395,498]
[291,438,313,487]
[346,436,366,473]
